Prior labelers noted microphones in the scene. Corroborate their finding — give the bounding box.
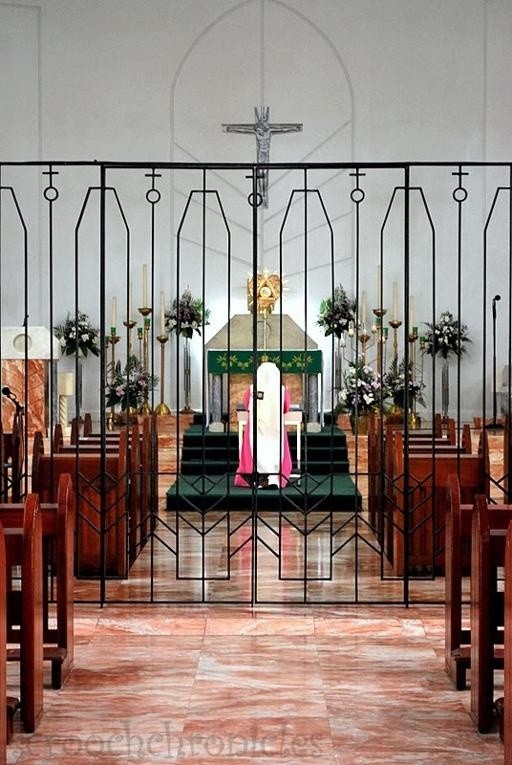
[2,387,21,409]
[493,295,501,316]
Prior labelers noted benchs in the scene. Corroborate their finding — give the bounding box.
[0,472,77,763]
[443,474,511,763]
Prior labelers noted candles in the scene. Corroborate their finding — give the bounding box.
[143,264,148,308]
[361,291,367,322]
[129,281,133,322]
[376,266,381,308]
[392,281,398,320]
[160,290,165,336]
[111,295,116,326]
[409,296,413,332]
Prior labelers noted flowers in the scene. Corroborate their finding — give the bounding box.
[316,290,355,341]
[380,358,427,400]
[166,291,209,338]
[56,313,101,361]
[426,313,465,359]
[338,356,382,411]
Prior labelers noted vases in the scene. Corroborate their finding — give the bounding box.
[351,412,369,434]
[182,337,192,412]
[334,342,342,406]
[393,392,413,409]
[441,360,449,418]
[72,356,86,424]
